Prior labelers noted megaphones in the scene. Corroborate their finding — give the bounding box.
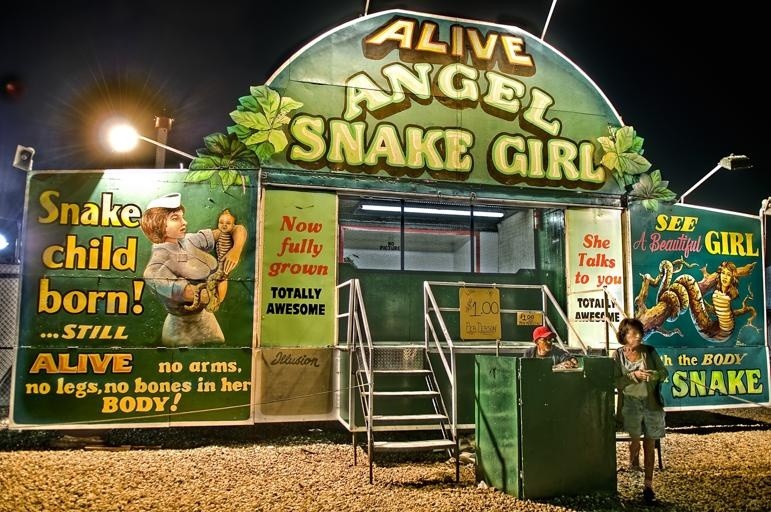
[11,144,36,172]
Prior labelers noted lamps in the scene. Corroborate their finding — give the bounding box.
[677,153,753,204]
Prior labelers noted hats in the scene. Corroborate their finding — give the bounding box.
[533,327,557,342]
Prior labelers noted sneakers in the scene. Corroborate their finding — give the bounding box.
[641,487,656,505]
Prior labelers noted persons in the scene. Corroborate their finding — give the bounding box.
[138,191,249,347]
[612,317,670,502]
[715,259,740,299]
[202,209,236,313]
[524,326,579,370]
